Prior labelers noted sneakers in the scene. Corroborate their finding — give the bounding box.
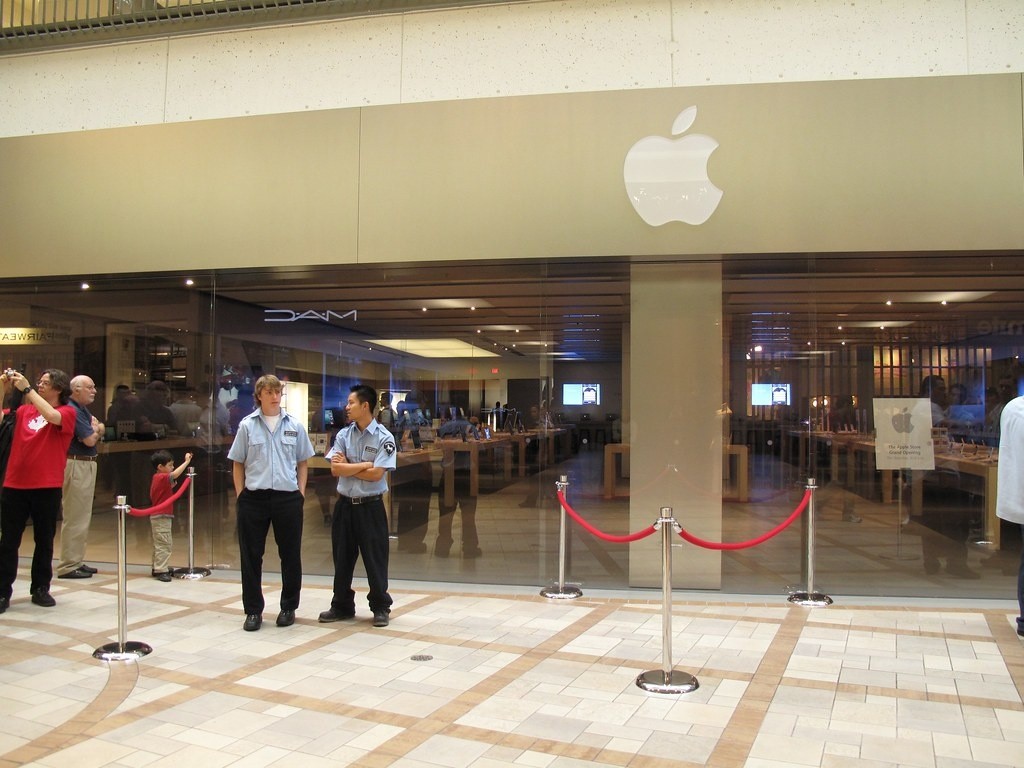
[0,596,10,615]
[32,586,56,607]
[318,603,356,623]
[434,547,448,558]
[276,605,295,625]
[243,609,263,631]
[464,548,481,558]
[374,607,389,626]
[398,540,427,553]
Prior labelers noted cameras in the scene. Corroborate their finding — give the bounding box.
[7,369,17,376]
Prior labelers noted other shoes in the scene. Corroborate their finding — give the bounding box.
[846,514,862,523]
[152,566,174,581]
[324,515,333,528]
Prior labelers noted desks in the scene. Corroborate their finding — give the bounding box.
[786,431,1000,551]
[308,425,566,534]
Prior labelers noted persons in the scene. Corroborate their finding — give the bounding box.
[435,392,486,559]
[378,393,395,428]
[814,406,862,523]
[227,374,315,632]
[521,408,545,507]
[150,450,192,582]
[168,382,227,555]
[389,391,432,555]
[920,374,1022,577]
[0,368,77,614]
[994,395,1024,636]
[107,385,130,524]
[131,381,180,545]
[56,374,105,581]
[319,385,397,627]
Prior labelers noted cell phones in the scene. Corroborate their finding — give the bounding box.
[460,428,491,443]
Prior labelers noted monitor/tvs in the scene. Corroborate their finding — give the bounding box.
[104,427,117,442]
[324,410,334,426]
[562,383,601,406]
[752,383,791,405]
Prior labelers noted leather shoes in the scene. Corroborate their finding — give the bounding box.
[58,570,92,579]
[79,563,98,575]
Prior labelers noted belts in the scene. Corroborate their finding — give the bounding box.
[337,494,382,505]
[66,452,98,460]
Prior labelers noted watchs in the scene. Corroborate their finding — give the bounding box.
[21,386,33,395]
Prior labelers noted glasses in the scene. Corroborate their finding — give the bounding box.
[36,381,51,387]
[78,385,95,390]
